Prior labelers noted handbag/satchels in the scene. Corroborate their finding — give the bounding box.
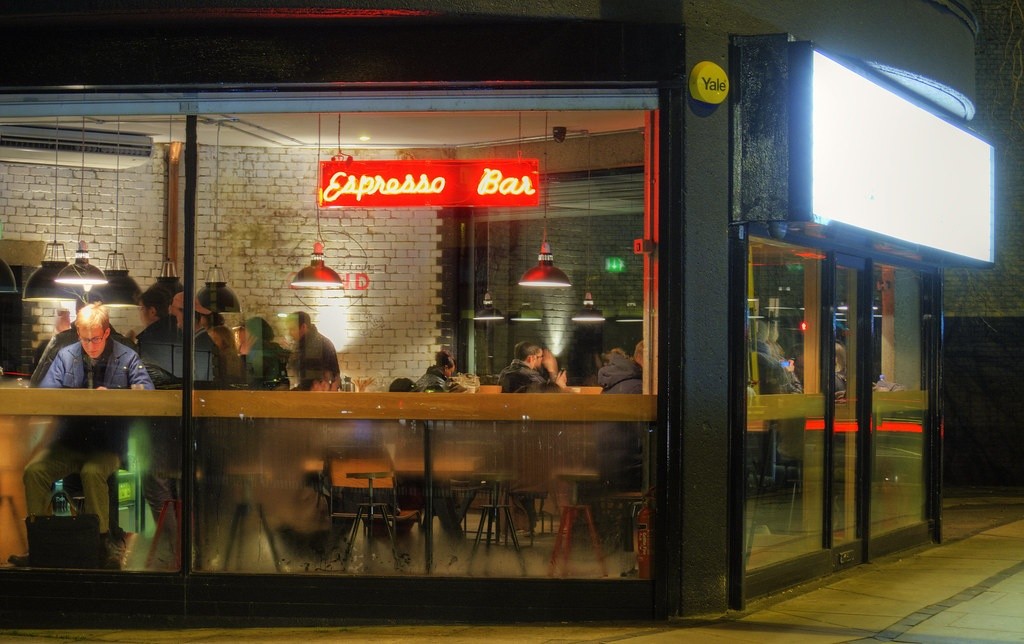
[447,372,480,393]
[25,492,102,569]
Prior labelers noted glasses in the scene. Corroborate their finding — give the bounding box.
[536,355,544,361]
[78,334,104,344]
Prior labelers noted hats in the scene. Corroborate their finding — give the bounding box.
[172,292,211,315]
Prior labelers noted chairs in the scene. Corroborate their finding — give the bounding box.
[59,385,647,579]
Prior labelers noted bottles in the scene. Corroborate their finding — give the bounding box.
[877,375,887,392]
[339,376,355,393]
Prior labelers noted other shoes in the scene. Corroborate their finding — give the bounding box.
[6,553,29,568]
[102,534,128,571]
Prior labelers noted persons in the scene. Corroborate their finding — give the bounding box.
[8,289,342,567]
[409,348,458,396]
[749,319,849,403]
[498,340,644,530]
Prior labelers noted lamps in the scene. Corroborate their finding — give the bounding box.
[21,116,85,302]
[514,111,573,289]
[510,208,543,321]
[141,113,184,315]
[289,113,344,287]
[472,222,504,320]
[614,173,644,321]
[0,258,19,292]
[571,137,605,322]
[93,94,142,308]
[196,123,242,314]
[55,86,109,286]
